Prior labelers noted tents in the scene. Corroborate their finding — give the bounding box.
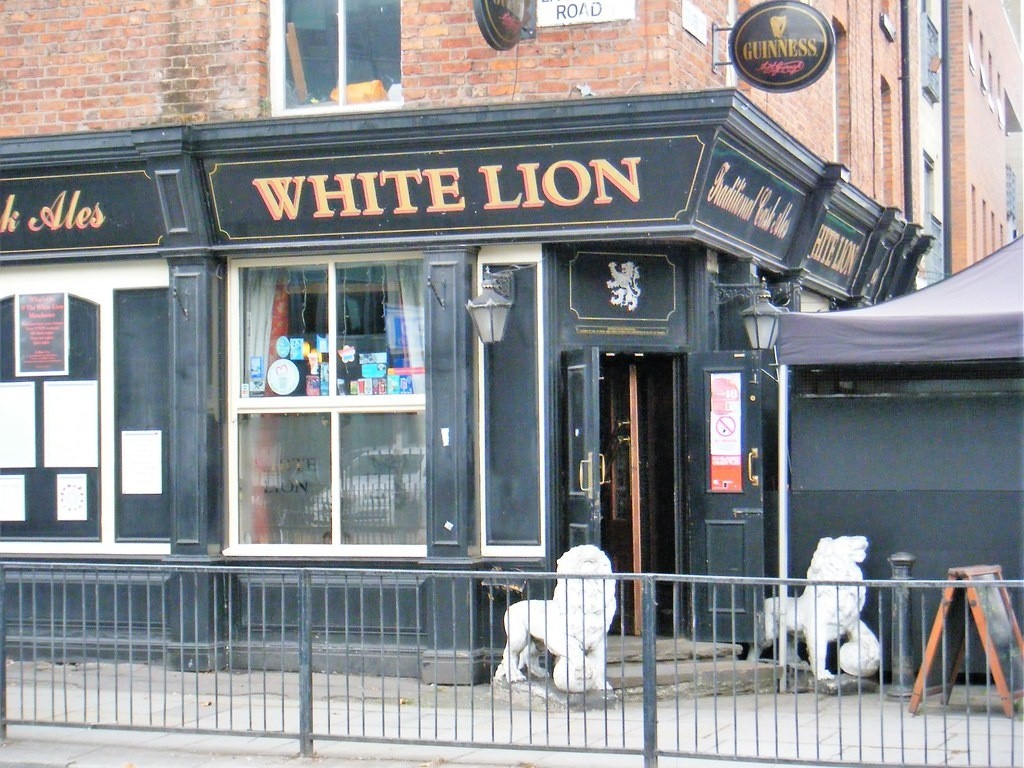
[777,232,1024,696]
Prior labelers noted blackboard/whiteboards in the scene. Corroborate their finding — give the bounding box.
[912,565,1024,701]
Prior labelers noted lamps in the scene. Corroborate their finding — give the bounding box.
[466,266,511,345]
